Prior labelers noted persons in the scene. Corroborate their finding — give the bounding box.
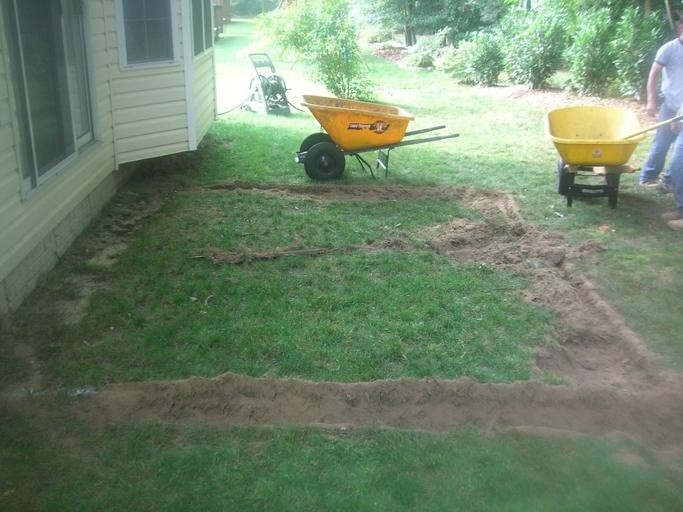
[636,20,683,189]
[660,104,683,232]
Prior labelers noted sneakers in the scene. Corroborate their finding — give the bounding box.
[662,208,683,233]
[639,179,660,190]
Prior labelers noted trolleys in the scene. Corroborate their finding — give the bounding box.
[545,104,646,208]
[241,51,290,116]
[295,93,459,181]
